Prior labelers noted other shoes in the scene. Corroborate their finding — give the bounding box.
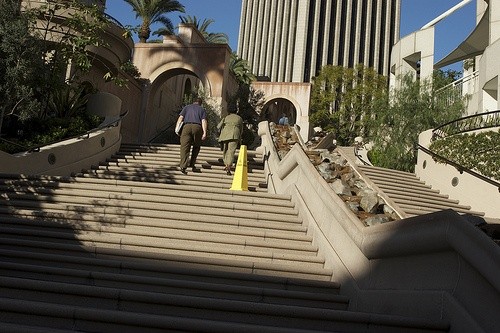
[180,168,185,173]
[224,167,232,176]
[188,163,196,168]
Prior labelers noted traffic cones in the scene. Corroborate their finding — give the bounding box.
[229,144,249,193]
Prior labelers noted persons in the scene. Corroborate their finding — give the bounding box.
[174,98,207,173]
[216,103,244,175]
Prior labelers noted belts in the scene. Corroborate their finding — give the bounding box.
[184,122,202,126]
